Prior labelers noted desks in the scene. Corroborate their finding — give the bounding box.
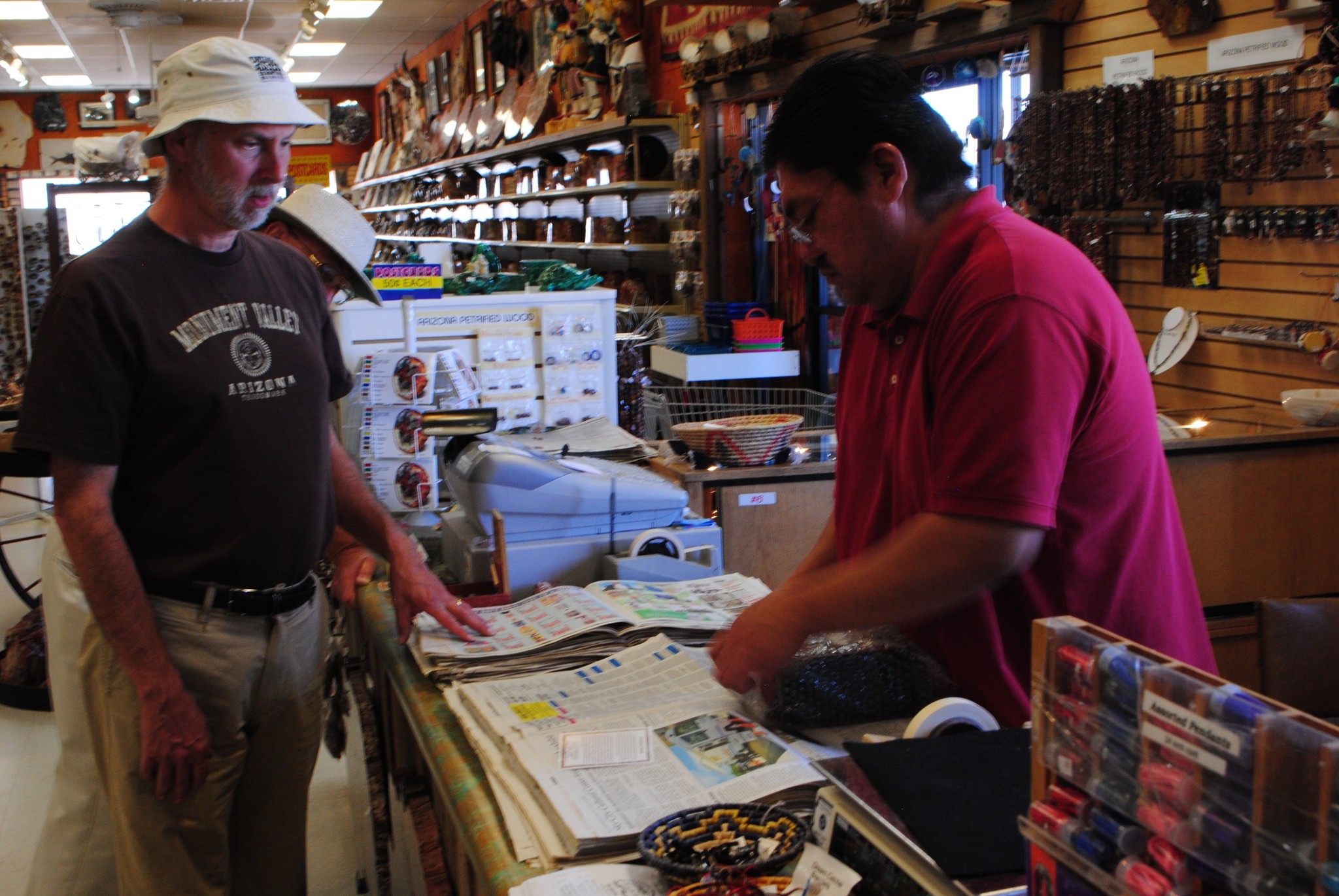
[640,402,1339,642]
[344,572,913,896]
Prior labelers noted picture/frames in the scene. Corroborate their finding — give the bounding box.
[76,99,116,130]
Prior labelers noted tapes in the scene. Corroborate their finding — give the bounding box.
[903,696,1000,738]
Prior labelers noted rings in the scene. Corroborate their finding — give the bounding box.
[457,599,462,607]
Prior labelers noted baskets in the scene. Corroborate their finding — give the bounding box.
[656,315,700,343]
[732,308,786,352]
[671,413,803,465]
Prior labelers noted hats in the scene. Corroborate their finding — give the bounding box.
[142,36,328,160]
[268,183,385,310]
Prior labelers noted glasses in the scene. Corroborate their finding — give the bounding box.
[789,169,848,248]
[283,225,358,307]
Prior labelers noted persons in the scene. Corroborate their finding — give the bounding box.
[710,51,1222,719]
[10,35,492,895]
[23,183,377,893]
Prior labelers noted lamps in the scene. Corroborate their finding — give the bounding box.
[299,0,330,36]
[100,85,116,104]
[6,58,23,75]
[127,87,140,103]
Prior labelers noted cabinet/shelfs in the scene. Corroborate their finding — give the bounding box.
[350,116,685,317]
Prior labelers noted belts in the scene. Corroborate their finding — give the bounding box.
[141,568,317,617]
